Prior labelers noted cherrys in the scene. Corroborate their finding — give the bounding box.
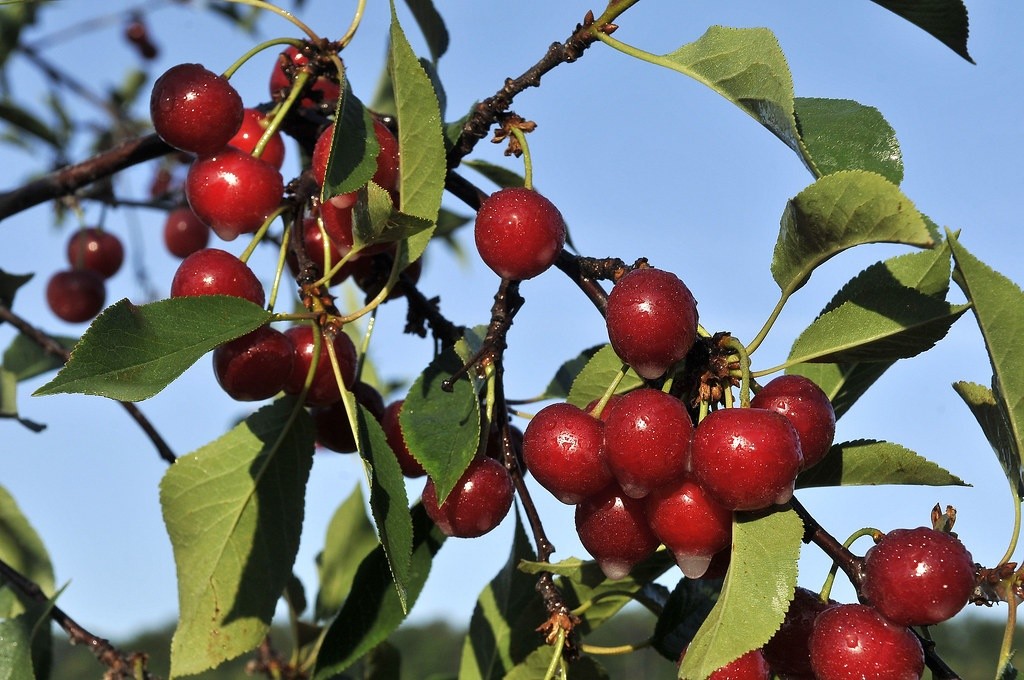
[45,38,974,680]
